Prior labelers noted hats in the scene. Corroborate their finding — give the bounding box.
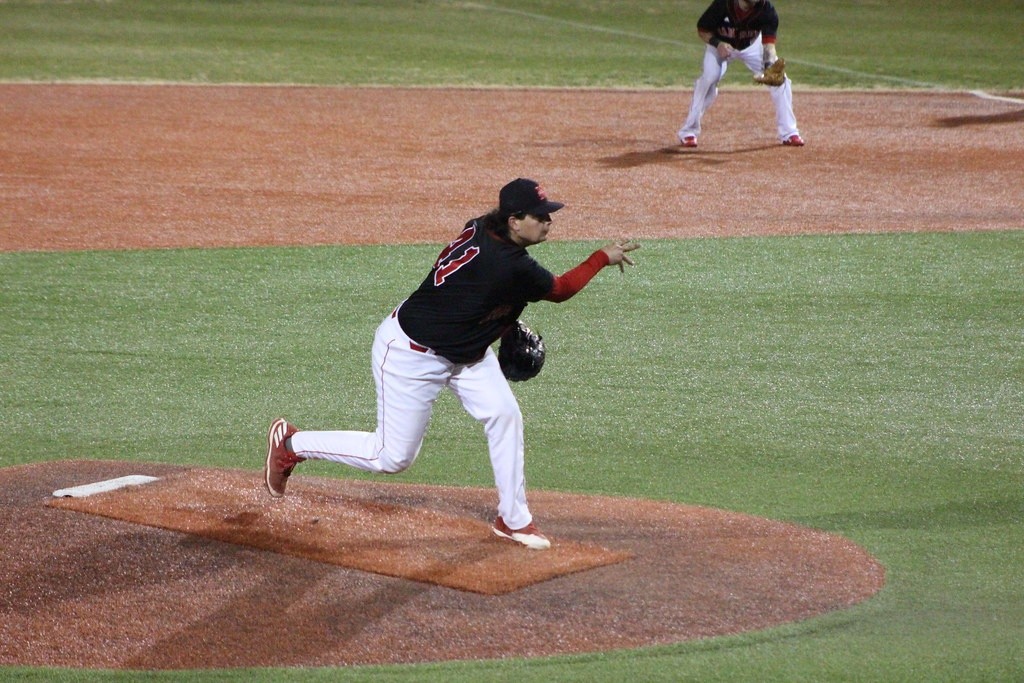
[499,177,564,217]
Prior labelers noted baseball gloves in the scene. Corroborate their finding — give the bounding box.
[498,320,546,383]
[752,57,786,87]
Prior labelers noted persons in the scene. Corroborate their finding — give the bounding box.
[677,0,805,147]
[265,177,641,549]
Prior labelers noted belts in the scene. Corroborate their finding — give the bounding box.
[392,307,440,355]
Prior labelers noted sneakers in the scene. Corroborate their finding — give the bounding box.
[783,135,804,146]
[681,136,698,146]
[264,418,307,498]
[493,516,551,550]
